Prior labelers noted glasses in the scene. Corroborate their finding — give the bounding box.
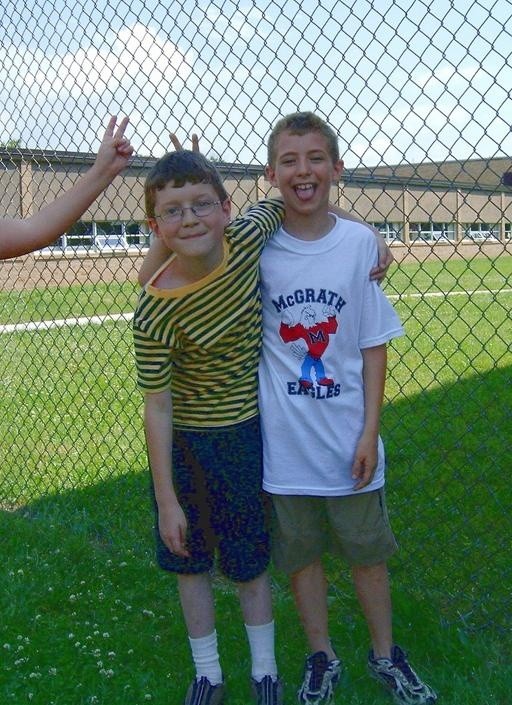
[151,199,227,224]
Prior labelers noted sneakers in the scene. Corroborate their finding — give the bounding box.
[365,642,438,705]
[183,672,229,705]
[296,650,344,705]
[248,671,284,704]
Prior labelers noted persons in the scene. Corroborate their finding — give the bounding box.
[133,150,396,704]
[1,116,133,262]
[140,112,446,704]
